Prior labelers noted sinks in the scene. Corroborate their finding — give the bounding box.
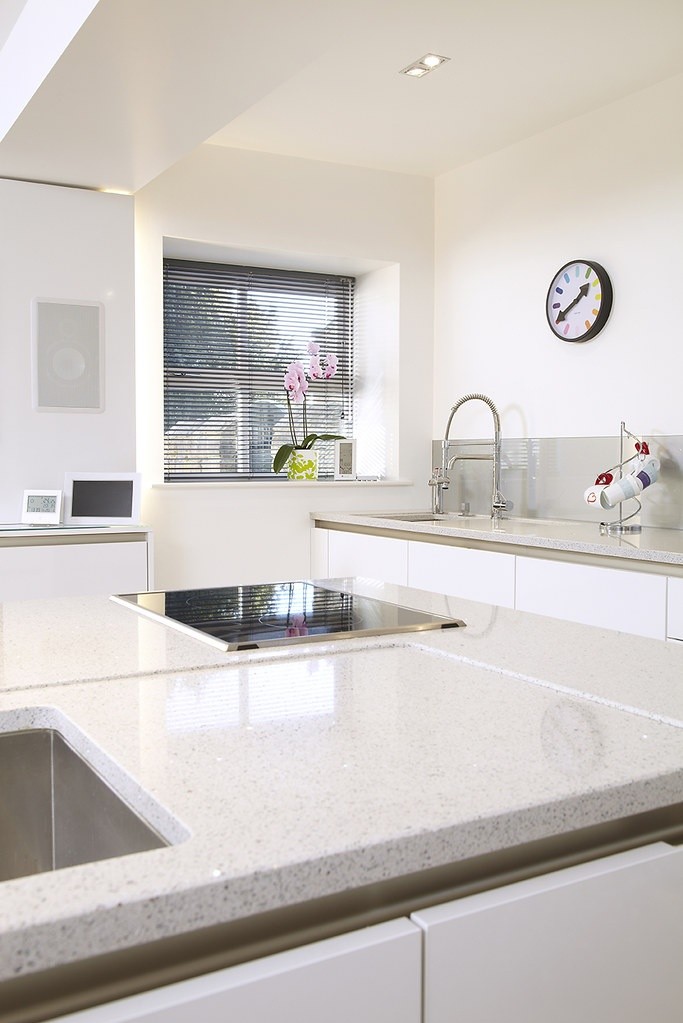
[348,511,488,523]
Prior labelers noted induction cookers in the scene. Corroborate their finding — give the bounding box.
[109,581,467,651]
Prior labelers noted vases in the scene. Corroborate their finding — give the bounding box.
[287,448,319,482]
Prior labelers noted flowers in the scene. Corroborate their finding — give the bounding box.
[282,337,336,404]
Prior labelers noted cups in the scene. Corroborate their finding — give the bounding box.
[632,454,660,471]
[584,473,616,510]
[601,470,640,506]
[630,459,657,491]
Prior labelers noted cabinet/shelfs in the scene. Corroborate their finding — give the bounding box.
[313,519,683,649]
[0,800,683,1023]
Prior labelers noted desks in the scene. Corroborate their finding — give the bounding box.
[0,524,154,601]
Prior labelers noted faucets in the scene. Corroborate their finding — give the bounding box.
[447,454,495,470]
[441,393,514,520]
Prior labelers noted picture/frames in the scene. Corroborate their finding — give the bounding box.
[30,295,107,414]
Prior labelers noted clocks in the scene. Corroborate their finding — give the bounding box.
[545,260,614,345]
